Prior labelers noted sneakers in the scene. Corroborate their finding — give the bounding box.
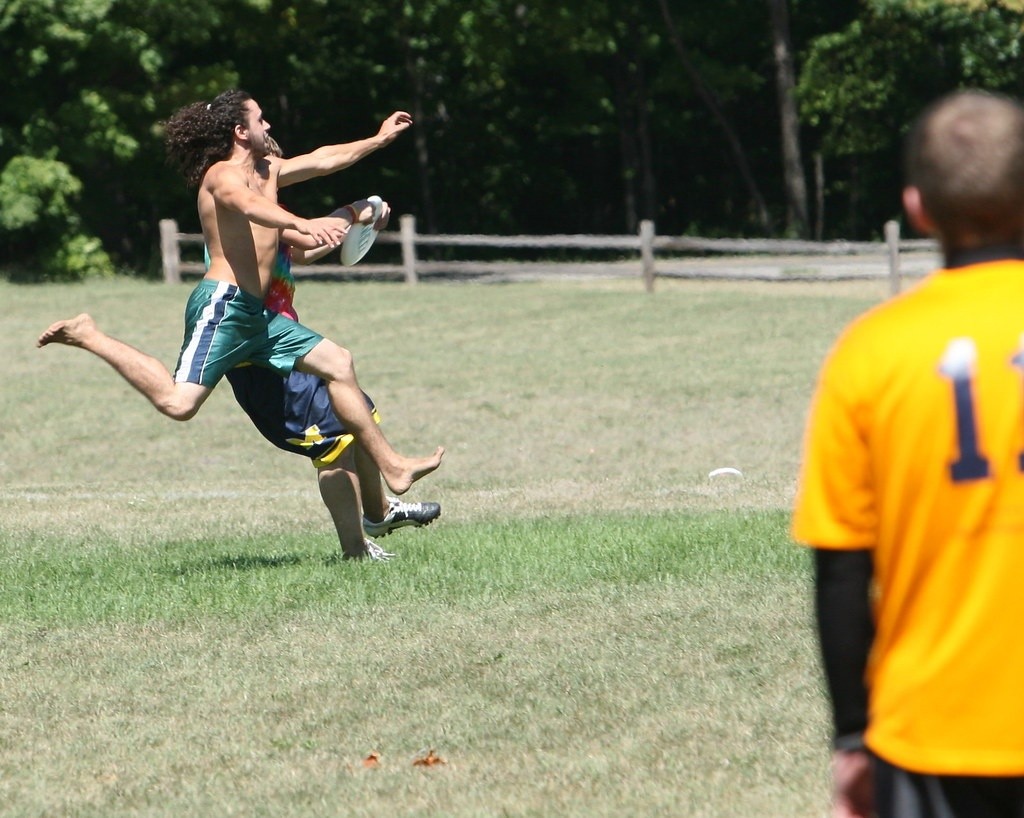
[340,538,396,566]
[362,496,441,539]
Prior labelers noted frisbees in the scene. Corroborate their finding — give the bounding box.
[340,194,384,267]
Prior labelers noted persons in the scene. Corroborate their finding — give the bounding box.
[35,89,444,495]
[784,88,1024,818]
[205,137,441,558]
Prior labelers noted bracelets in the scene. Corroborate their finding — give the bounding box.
[343,204,360,225]
